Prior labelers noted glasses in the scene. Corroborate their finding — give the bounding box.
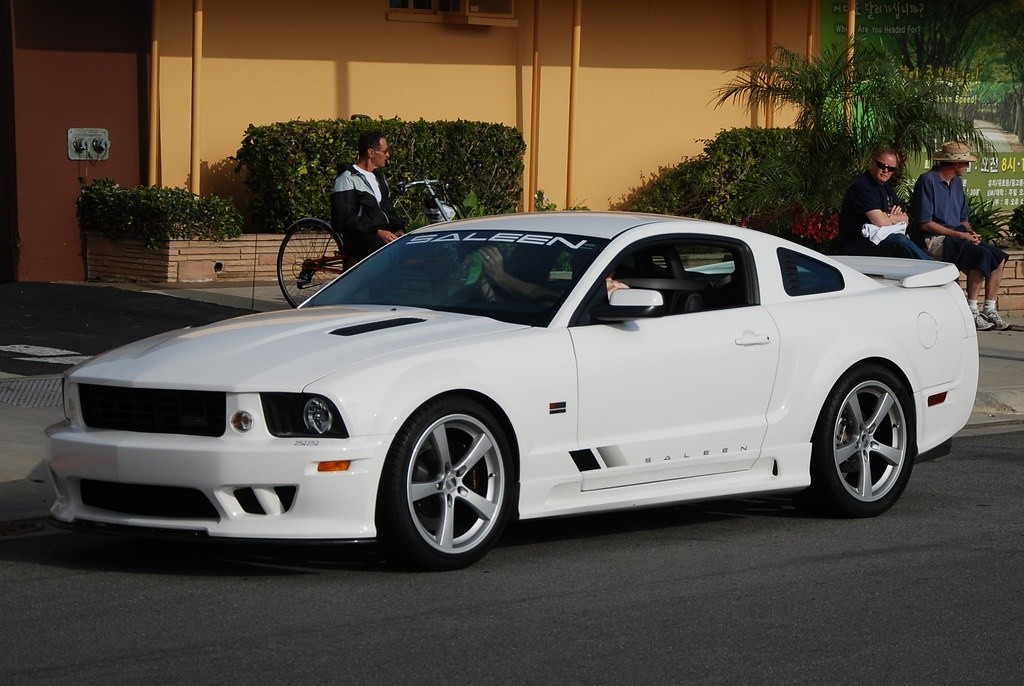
[875,159,896,172]
[373,148,388,155]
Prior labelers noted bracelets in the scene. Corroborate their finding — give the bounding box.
[887,212,890,218]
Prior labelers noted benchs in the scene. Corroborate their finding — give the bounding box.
[651,266,757,306]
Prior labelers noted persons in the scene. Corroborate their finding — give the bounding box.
[475,243,631,309]
[330,130,405,274]
[842,142,1011,331]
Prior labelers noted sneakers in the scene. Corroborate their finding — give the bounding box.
[972,313,995,330]
[983,310,1012,331]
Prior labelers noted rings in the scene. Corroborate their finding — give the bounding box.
[484,256,490,260]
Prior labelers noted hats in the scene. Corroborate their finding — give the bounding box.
[932,141,977,163]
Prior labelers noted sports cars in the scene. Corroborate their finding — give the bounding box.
[41,211,980,572]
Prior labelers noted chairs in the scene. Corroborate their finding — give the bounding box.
[661,287,704,318]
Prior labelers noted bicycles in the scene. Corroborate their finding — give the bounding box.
[276,177,461,310]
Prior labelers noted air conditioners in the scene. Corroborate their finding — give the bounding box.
[446,1,516,20]
